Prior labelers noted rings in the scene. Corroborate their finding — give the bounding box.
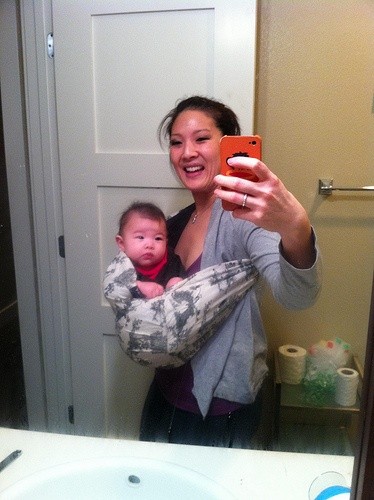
[242,194,248,208]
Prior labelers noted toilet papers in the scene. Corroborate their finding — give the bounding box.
[332,367,359,406]
[272,344,308,384]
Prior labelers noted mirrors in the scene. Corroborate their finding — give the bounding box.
[0,0,374,500]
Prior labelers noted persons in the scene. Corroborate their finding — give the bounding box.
[139,97,323,449]
[103,201,234,370]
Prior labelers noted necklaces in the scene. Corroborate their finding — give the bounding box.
[192,202,212,224]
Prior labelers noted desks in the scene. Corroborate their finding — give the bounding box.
[274,382,361,437]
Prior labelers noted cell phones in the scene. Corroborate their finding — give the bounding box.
[218,134,262,212]
